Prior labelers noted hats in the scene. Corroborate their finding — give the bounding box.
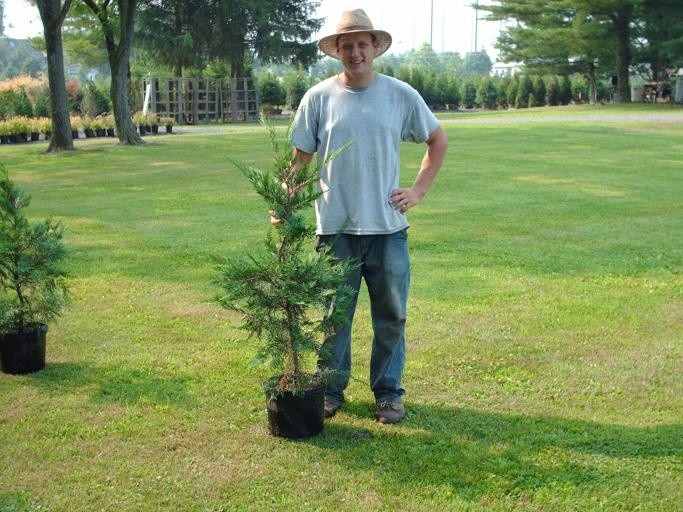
[317,9,392,58]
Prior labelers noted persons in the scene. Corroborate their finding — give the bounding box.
[266,8,448,423]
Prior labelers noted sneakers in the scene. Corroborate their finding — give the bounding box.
[376,399,404,423]
[324,399,339,416]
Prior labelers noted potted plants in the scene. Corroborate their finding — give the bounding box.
[379,64,589,111]
[0,111,180,145]
[0,161,78,377]
[192,106,368,439]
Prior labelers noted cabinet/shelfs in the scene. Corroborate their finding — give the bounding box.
[141,77,259,124]
